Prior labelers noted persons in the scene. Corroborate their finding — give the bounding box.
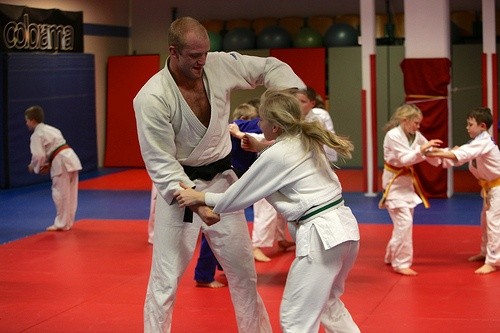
[377,102,458,277]
[25,107,81,231]
[174,89,360,333]
[194,86,337,287]
[424,106,500,275]
[132,17,306,333]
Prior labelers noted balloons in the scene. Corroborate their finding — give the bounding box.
[289,27,324,48]
[222,25,256,51]
[208,29,221,51]
[258,28,293,47]
[324,23,357,52]
